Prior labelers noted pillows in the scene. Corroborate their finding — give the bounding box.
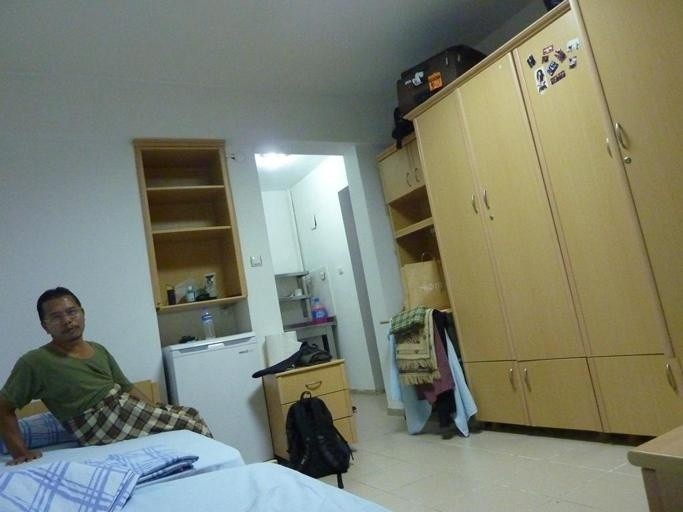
[0,410,79,455]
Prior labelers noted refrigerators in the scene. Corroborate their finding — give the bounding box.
[161,332,274,465]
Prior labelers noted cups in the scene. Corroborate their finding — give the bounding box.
[166,289,176,305]
[295,289,303,296]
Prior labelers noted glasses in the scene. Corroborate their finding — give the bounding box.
[44,306,81,324]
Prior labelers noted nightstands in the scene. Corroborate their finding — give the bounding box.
[263,358,357,461]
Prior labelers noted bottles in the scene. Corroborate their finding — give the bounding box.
[200,306,217,340]
[186,286,196,302]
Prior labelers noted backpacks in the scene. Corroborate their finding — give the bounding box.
[285,391,354,479]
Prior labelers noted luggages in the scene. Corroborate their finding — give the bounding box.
[396,44,488,118]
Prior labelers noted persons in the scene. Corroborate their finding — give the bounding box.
[0,286,213,468]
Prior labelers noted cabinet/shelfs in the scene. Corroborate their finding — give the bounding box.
[401,43,603,434]
[131,137,249,314]
[510,1,682,439]
[377,130,441,265]
[274,270,313,327]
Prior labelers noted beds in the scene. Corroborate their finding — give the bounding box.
[0,379,391,512]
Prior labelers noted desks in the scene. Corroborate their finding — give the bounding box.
[627,425,683,512]
[283,320,338,360]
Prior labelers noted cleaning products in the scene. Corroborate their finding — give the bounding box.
[205,273,217,298]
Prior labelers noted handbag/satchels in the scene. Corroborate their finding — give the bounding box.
[400,252,451,313]
